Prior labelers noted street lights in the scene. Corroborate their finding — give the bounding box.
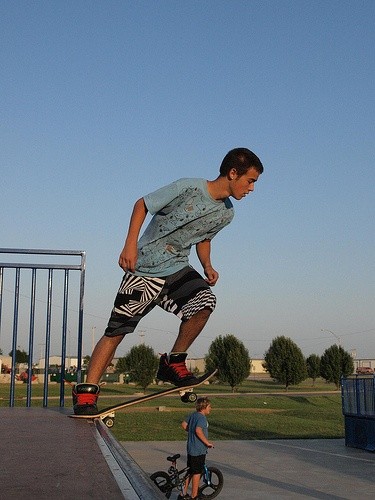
[321,327,340,391]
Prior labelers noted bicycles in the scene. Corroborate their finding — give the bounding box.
[149,446,224,499]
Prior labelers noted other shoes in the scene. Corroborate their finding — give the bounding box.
[177,493,214,500]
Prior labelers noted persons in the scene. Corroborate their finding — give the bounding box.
[180,397,214,500]
[72,147,266,416]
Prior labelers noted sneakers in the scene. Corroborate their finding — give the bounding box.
[71,383,99,415]
[157,352,199,385]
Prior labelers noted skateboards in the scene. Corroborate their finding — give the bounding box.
[67,367,218,430]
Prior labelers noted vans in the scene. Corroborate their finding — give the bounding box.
[356,367,374,374]
